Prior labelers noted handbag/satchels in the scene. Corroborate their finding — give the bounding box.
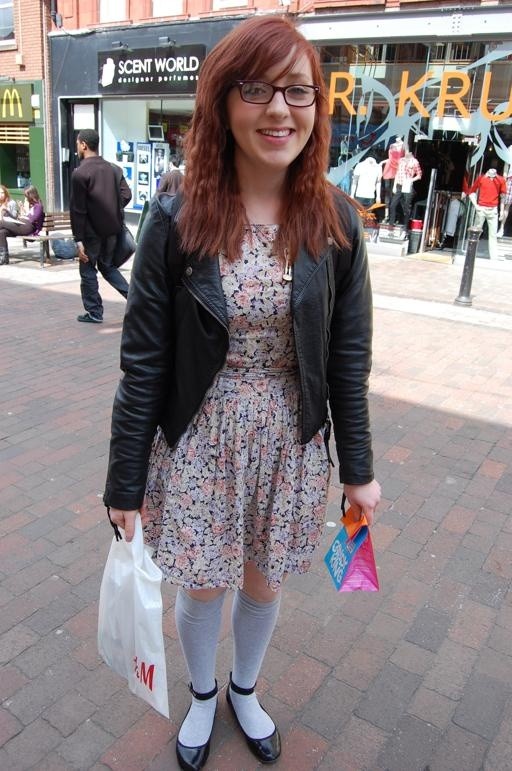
[113,223,135,268]
[97,514,169,723]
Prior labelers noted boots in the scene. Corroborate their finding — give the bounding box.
[0,248,9,265]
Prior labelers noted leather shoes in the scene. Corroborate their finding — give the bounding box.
[176,677,219,771]
[225,671,282,763]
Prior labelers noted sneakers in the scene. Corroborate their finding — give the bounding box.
[77,312,103,323]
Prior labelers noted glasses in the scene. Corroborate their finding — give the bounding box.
[233,80,321,107]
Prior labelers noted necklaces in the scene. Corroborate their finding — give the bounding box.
[246,217,277,245]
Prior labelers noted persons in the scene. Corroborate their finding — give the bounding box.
[101,14,382,770]
[68,128,132,324]
[496,145,512,239]
[1,184,18,220]
[1,185,45,266]
[469,168,507,261]
[339,135,423,227]
[158,154,185,195]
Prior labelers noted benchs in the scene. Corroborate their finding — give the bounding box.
[15,211,76,270]
